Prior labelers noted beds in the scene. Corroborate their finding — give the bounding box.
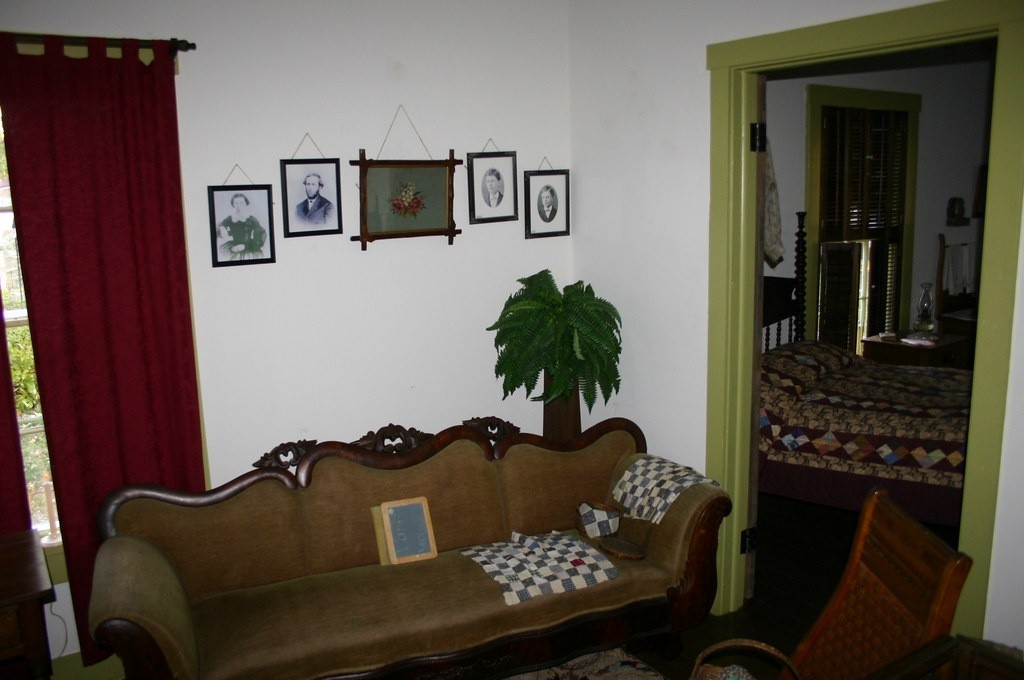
[756,213,975,552]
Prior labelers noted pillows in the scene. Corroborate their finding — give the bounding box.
[759,339,876,402]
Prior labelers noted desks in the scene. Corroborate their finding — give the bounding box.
[0,528,57,680]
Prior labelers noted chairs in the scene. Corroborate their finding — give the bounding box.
[779,487,973,680]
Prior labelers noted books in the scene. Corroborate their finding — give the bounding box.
[879,333,897,341]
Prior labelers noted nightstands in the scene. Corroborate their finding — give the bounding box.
[860,330,971,368]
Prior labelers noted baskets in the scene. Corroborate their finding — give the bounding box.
[691,639,801,680]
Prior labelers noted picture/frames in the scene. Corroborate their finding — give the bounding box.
[524,169,571,240]
[466,151,519,225]
[207,184,276,268]
[280,158,343,238]
[382,497,438,565]
[348,149,463,252]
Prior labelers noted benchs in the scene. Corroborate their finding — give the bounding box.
[87,416,732,680]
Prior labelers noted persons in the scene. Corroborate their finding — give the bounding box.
[484,169,504,207]
[296,173,334,225]
[539,186,557,223]
[216,193,267,260]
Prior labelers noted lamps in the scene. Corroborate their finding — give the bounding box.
[912,280,937,332]
